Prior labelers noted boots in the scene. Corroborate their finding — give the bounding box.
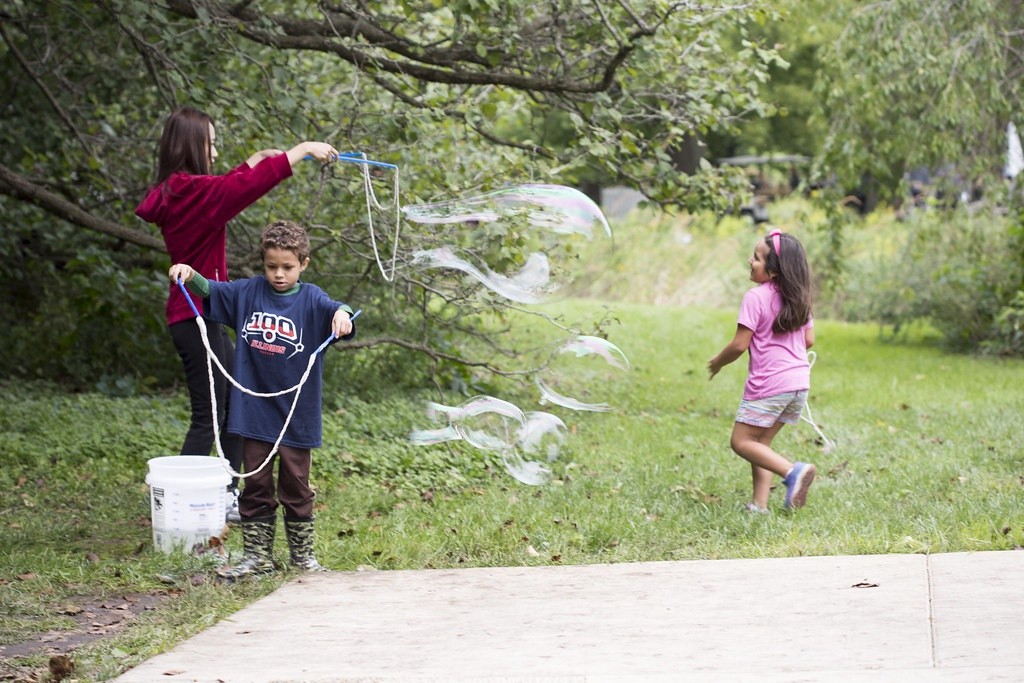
[215,521,278,579]
[284,517,327,572]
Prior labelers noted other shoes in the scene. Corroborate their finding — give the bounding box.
[781,463,814,508]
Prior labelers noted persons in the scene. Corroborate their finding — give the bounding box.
[135,107,339,522]
[705,229,817,513]
[169,221,356,580]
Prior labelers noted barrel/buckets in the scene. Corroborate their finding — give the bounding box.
[144,456,230,554]
